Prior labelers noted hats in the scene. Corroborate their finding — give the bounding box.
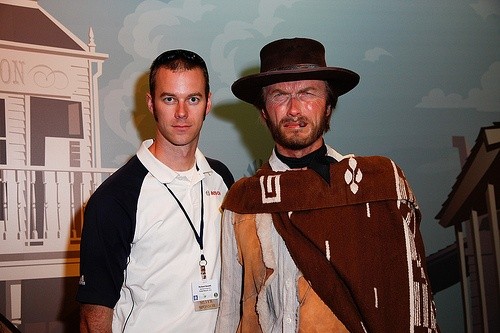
[231,37,360,108]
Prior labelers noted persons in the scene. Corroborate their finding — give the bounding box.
[79,50,235,333]
[215,38,439,333]
